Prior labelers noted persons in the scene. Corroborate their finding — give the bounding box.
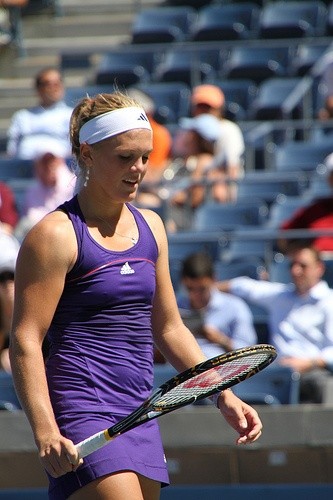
[8,93,263,500]
[0,66,333,405]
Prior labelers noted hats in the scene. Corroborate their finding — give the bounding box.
[191,84,225,108]
[179,113,222,139]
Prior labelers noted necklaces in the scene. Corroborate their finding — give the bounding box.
[85,205,138,245]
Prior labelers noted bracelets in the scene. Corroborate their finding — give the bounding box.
[308,358,319,367]
[213,388,233,409]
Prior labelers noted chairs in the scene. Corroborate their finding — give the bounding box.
[0,0,333,410]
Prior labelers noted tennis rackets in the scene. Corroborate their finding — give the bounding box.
[74,344,279,457]
